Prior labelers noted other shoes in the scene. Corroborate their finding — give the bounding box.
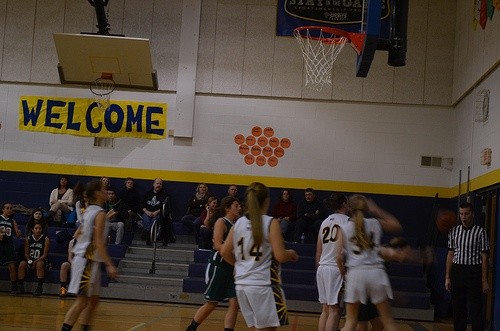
[33,286,42,296]
[10,284,25,295]
[60,287,67,297]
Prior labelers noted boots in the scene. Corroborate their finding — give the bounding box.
[46,210,55,221]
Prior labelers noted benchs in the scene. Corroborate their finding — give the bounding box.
[0,225,443,311]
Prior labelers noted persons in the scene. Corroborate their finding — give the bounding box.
[313,191,351,326]
[186,197,242,331]
[1,176,137,330]
[444,202,491,331]
[16,225,51,297]
[45,176,75,228]
[220,182,300,330]
[335,193,401,326]
[60,180,118,330]
[302,186,411,326]
[1,202,25,294]
[183,180,298,331]
[140,178,168,247]
